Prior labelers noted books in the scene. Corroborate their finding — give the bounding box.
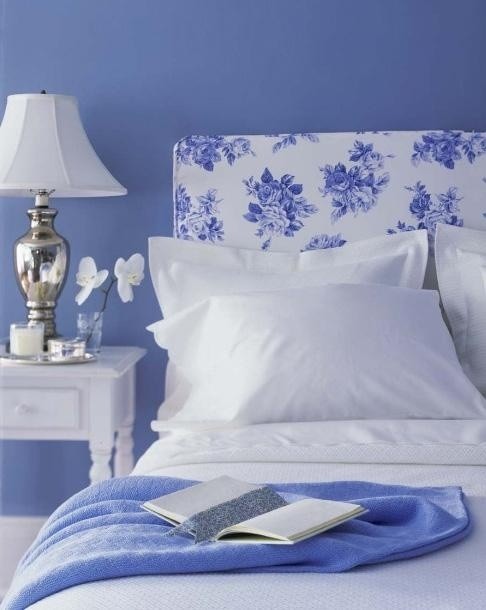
[140,474,369,545]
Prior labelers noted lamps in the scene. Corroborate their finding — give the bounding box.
[0,88,128,359]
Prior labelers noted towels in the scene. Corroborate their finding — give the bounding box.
[9,474,472,607]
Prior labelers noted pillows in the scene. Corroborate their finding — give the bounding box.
[434,225,486,396]
[148,229,428,320]
[145,285,486,431]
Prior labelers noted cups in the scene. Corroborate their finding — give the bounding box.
[9,321,45,358]
[47,339,74,363]
[66,337,87,359]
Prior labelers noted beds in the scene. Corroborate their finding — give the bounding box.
[8,130,486,607]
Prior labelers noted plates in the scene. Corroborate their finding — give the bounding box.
[1,349,96,368]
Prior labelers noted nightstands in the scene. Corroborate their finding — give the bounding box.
[0,345,146,490]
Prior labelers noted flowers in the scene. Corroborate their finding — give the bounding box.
[74,249,145,343]
